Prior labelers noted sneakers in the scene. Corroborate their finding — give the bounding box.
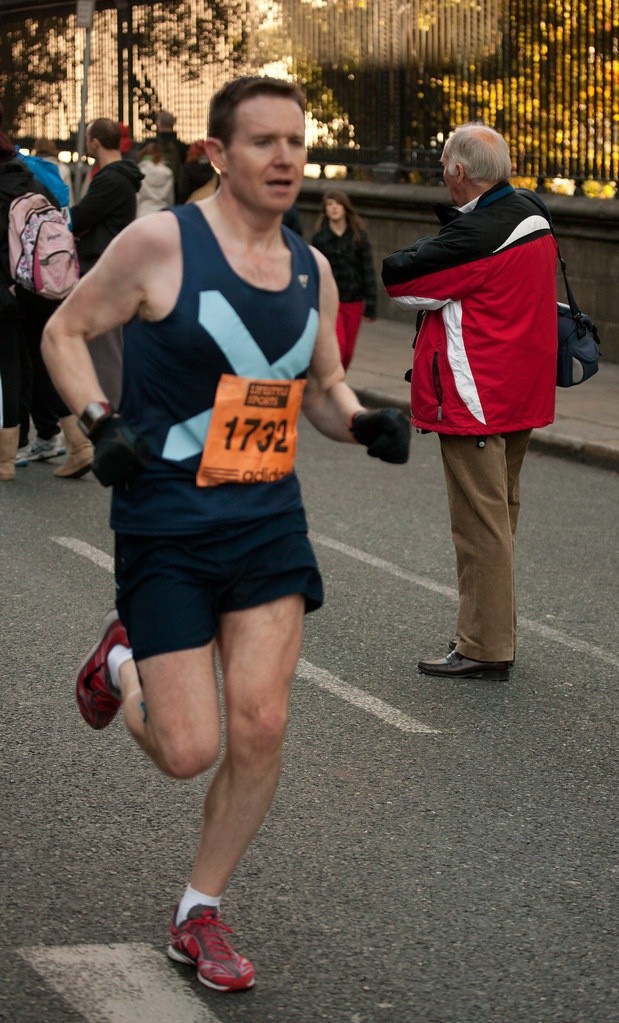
[167,904,256,993]
[16,434,65,466]
[74,609,132,730]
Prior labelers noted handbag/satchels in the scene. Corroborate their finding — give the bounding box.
[516,189,603,387]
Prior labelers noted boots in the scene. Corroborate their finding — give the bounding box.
[53,415,94,478]
[0,425,20,481]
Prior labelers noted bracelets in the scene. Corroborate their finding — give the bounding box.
[76,401,112,436]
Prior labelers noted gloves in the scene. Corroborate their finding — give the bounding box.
[349,407,411,464]
[86,411,172,487]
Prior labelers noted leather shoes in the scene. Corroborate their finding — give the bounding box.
[418,650,509,681]
[448,638,516,666]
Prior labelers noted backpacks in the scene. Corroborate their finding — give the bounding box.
[9,191,80,301]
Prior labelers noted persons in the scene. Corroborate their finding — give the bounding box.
[0,113,176,482]
[310,190,378,375]
[282,204,303,237]
[382,126,558,681]
[40,75,412,991]
[179,140,215,206]
